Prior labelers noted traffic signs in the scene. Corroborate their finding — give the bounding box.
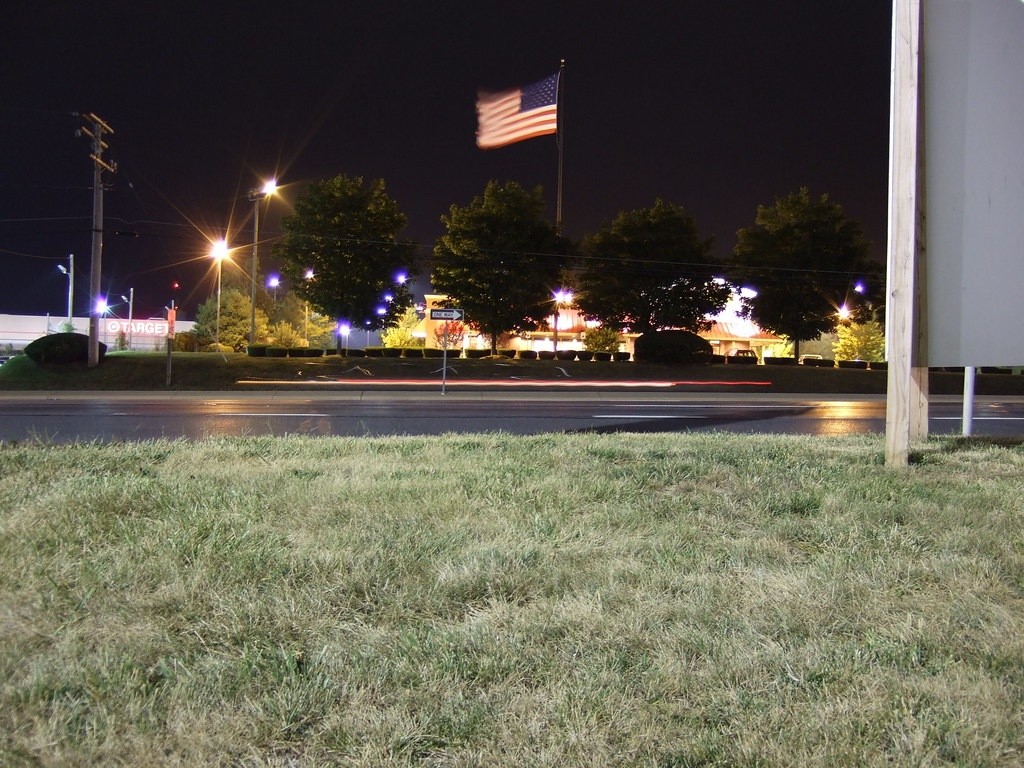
[429,307,465,321]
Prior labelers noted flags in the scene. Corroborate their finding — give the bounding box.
[476,71,560,148]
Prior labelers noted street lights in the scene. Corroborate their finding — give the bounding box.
[213,242,227,353]
[553,292,574,353]
[248,182,276,344]
[57,253,76,329]
